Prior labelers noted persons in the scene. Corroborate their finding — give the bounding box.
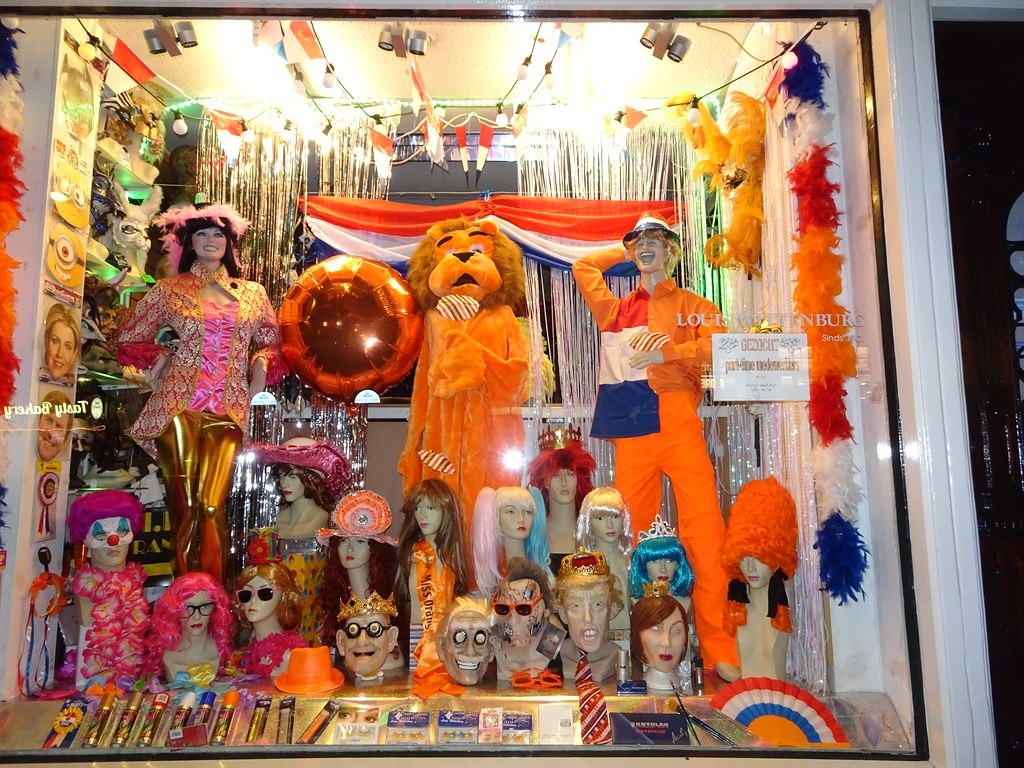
[38,302,81,386]
[339,705,380,724]
[75,208,793,698]
[33,389,75,459]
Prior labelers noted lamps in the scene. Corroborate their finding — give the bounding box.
[377,22,435,59]
[373,114,384,132]
[142,17,199,56]
[509,104,523,128]
[639,22,692,63]
[686,94,699,123]
[316,124,332,146]
[495,103,509,127]
[517,57,531,81]
[323,62,334,89]
[77,35,101,60]
[293,70,305,94]
[544,61,553,89]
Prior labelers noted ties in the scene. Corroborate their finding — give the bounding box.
[575,647,612,745]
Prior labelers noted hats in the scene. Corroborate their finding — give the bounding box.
[254,436,352,495]
[623,210,680,251]
[274,646,345,693]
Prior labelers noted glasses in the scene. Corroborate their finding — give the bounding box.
[509,668,562,688]
[178,602,217,619]
[342,622,390,638]
[491,589,545,616]
[236,584,276,606]
